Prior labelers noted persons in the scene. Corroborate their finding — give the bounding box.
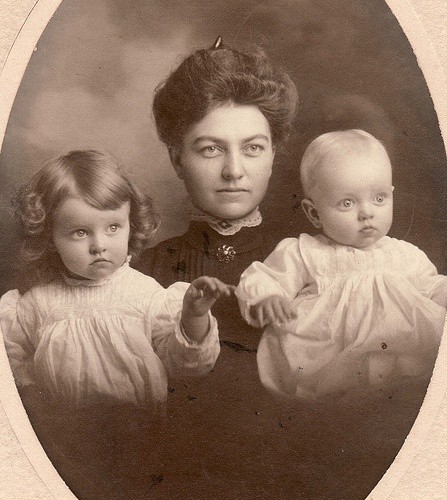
[132,33,302,353]
[1,150,231,409]
[236,128,447,394]
